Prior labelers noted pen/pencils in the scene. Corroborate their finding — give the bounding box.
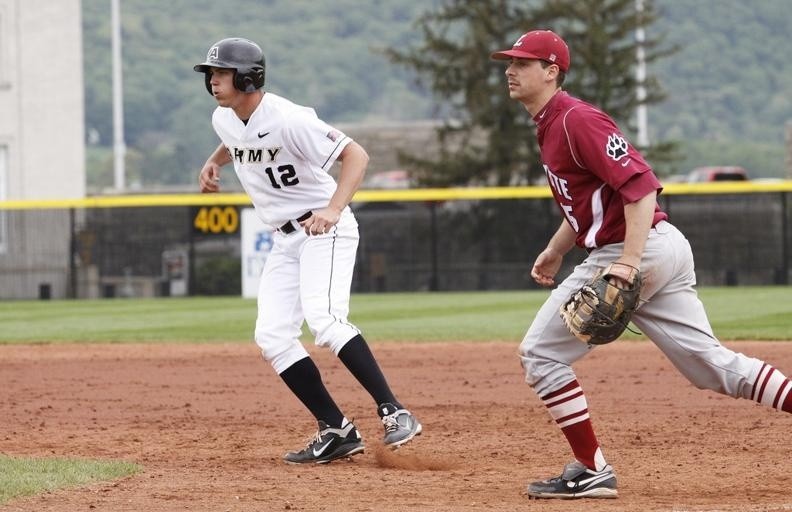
[686,166,747,182]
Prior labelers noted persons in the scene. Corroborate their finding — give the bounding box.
[485,24,792,503]
[188,33,425,467]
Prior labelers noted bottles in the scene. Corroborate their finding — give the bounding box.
[489,30,570,72]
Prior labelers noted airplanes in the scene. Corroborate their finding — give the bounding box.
[561,259,641,347]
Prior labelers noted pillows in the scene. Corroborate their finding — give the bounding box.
[194,38,266,98]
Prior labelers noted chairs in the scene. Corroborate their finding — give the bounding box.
[376,402,424,451]
[523,460,620,502]
[284,419,364,464]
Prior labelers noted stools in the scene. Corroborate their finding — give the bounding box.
[276,212,315,236]
